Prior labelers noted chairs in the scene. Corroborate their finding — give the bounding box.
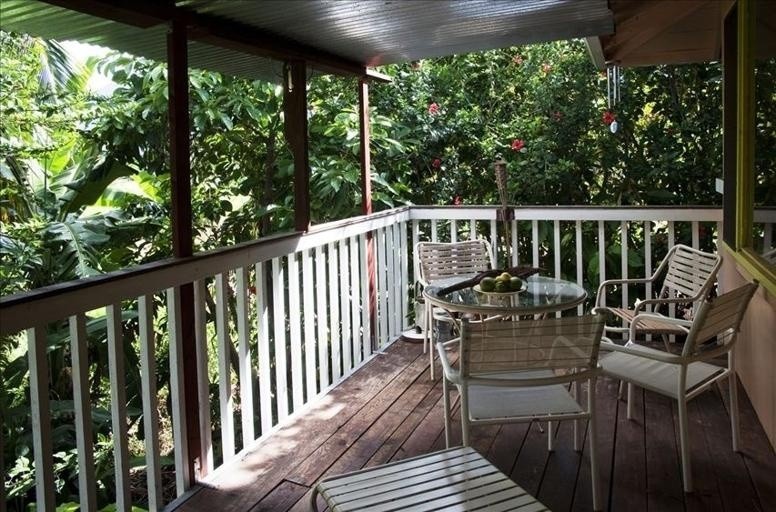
[308,445,552,512]
[573,277,762,493]
[415,238,500,382]
[592,246,726,422]
[436,307,610,512]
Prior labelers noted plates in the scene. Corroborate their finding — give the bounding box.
[473,283,527,295]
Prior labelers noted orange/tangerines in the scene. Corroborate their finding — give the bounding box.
[480,272,522,292]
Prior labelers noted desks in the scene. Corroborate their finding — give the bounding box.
[422,275,589,435]
